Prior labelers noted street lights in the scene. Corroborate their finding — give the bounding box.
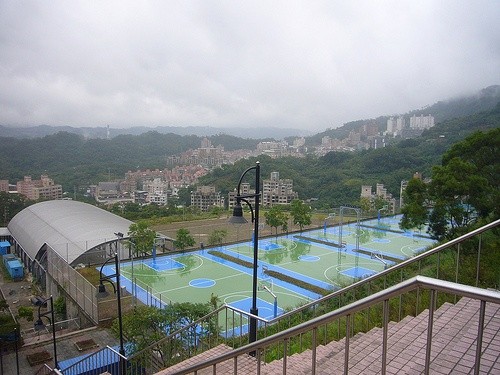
[94,252,128,375]
[229,160,266,358]
[33,295,61,375]
[2,328,21,375]
